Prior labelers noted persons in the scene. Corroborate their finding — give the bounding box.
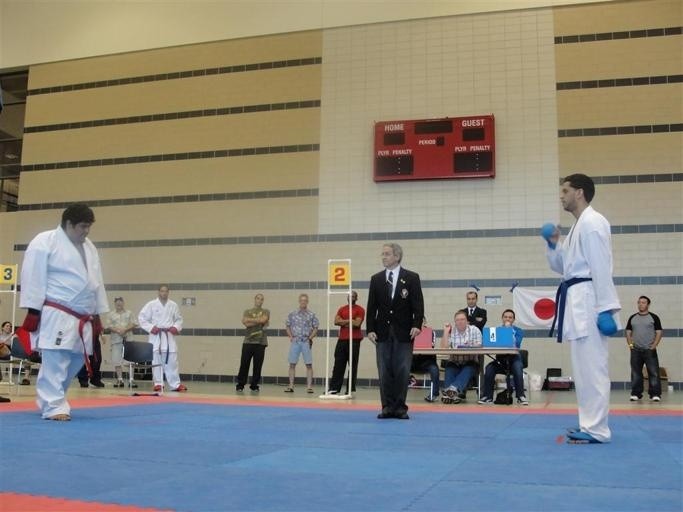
[138,286,188,391]
[284,294,319,393]
[236,294,270,392]
[0,313,104,403]
[323,291,365,395]
[20,203,110,421]
[441,312,482,404]
[458,292,487,400]
[477,310,528,405]
[541,174,620,444]
[107,297,138,388]
[408,315,440,402]
[366,243,424,420]
[625,296,663,402]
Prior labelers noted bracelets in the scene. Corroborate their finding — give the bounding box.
[629,344,633,347]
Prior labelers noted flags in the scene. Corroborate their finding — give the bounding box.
[513,288,559,330]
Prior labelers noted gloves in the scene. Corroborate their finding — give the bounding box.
[597,310,618,336]
[23,307,42,332]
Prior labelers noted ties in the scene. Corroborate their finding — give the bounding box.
[386,271,394,298]
[470,308,474,315]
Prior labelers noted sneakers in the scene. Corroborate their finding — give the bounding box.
[424,392,439,402]
[407,375,417,388]
[650,395,661,401]
[306,388,314,393]
[377,411,395,419]
[237,386,243,392]
[154,383,164,391]
[114,382,124,388]
[22,378,31,385]
[441,387,462,405]
[477,395,493,405]
[630,394,639,401]
[284,388,294,392]
[170,384,187,392]
[252,386,259,392]
[128,382,138,388]
[394,410,410,420]
[517,395,529,405]
[80,379,104,388]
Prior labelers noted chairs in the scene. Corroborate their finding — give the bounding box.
[123,341,163,396]
[411,330,528,402]
[10,340,42,385]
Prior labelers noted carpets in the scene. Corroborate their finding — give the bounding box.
[0,403,683,512]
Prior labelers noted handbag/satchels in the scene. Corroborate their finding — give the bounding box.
[494,388,514,405]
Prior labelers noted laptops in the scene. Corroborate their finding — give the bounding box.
[482,327,516,347]
[413,328,432,348]
